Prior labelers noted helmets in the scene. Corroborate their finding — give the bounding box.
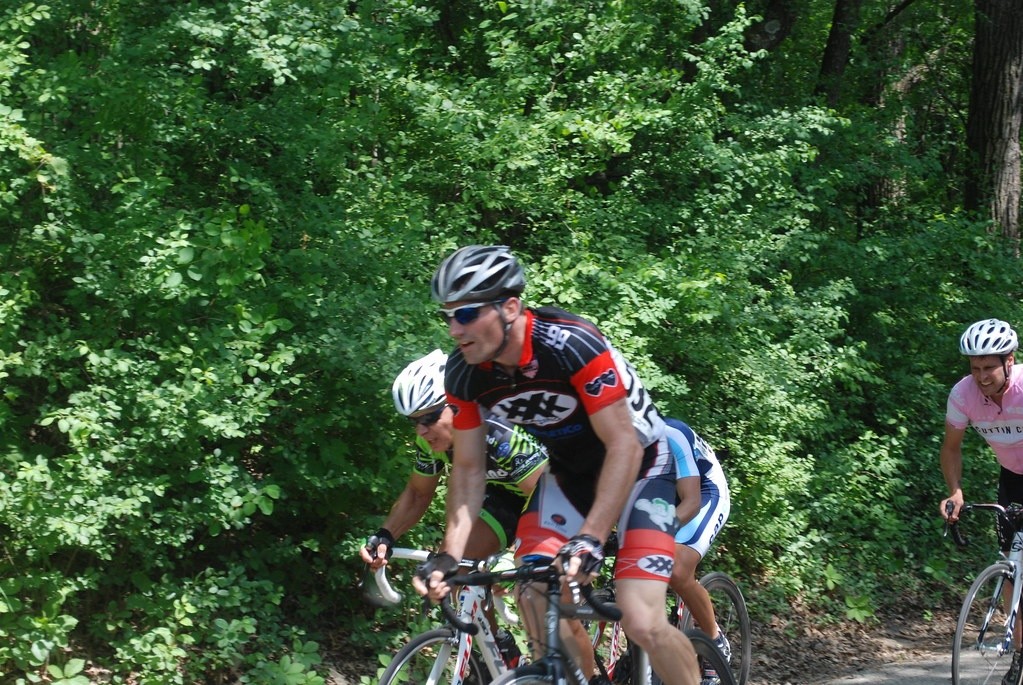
[392,349,450,417]
[431,244,526,301]
[959,318,1019,356]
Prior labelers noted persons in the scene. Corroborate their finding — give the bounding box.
[655,412,733,685]
[941,317,1023,685]
[407,243,701,685]
[358,350,547,675]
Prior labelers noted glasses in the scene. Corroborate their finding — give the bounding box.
[438,300,506,326]
[406,402,450,427]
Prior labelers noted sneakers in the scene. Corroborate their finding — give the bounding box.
[712,620,731,665]
[500,644,527,670]
[1000,648,1023,685]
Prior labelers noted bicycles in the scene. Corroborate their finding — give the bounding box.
[940,500,1023,685]
[369,540,690,685]
[434,539,751,684]
